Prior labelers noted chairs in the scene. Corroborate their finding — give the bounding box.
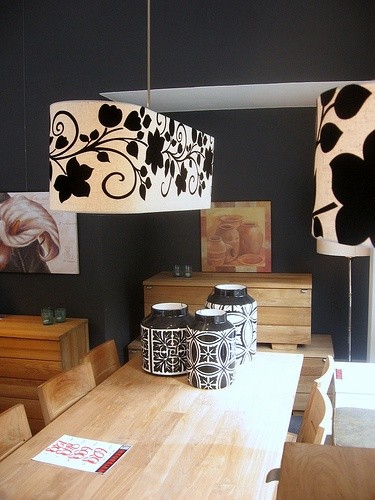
[285,355,334,445]
[0,339,122,462]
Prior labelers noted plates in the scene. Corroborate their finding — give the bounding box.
[238,254,264,265]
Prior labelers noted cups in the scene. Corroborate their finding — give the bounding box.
[40,304,54,325]
[172,264,181,277]
[55,308,66,323]
[183,265,192,278]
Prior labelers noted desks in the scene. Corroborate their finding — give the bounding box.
[0,346,305,500]
[142,271,312,351]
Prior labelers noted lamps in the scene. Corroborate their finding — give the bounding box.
[46,0,217,216]
[311,83,375,366]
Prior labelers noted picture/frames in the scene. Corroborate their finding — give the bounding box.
[198,199,272,275]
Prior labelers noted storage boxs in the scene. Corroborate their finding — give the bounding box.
[127,333,334,418]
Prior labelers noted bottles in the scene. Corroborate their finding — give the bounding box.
[140,302,192,377]
[204,283,258,367]
[207,214,264,266]
[186,309,237,390]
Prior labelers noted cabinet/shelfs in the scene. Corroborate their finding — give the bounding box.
[0,314,90,432]
